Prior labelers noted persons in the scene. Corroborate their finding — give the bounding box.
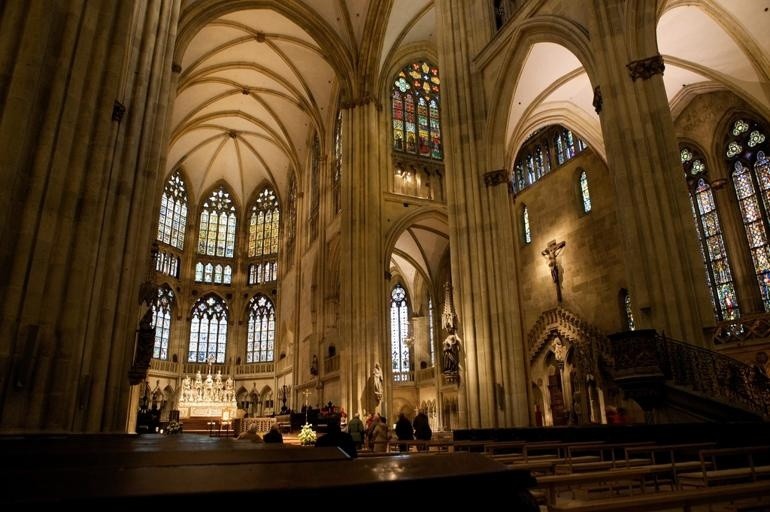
[371,361,384,394]
[349,411,392,452]
[263,425,284,443]
[609,406,633,425]
[395,411,414,452]
[414,411,432,450]
[314,413,358,459]
[236,422,262,442]
[441,325,459,375]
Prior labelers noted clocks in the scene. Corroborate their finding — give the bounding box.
[390,284,406,307]
